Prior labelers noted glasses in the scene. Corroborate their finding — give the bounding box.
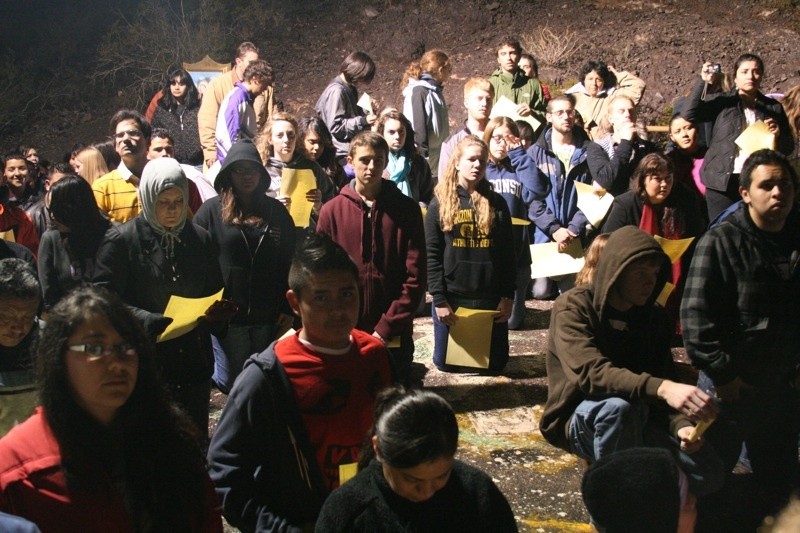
[492,135,504,143]
[65,341,130,357]
[231,165,259,176]
[113,130,140,141]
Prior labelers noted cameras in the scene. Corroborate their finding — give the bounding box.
[708,64,721,74]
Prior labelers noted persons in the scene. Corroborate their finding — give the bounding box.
[0,36,800,533]
[312,387,521,533]
